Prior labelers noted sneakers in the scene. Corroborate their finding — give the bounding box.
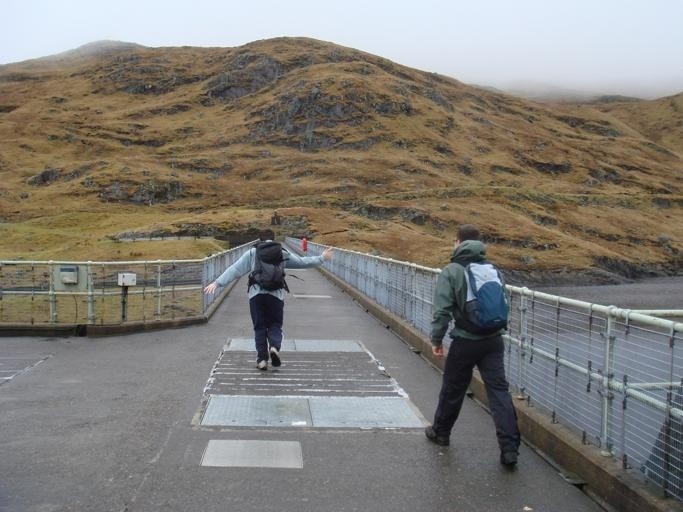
[425,424,452,447]
[269,346,281,368]
[256,359,269,371]
[499,449,520,465]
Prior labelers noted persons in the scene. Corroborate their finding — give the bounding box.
[202,229,334,370]
[425,225,520,466]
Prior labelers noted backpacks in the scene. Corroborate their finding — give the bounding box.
[247,241,288,293]
[453,257,511,338]
